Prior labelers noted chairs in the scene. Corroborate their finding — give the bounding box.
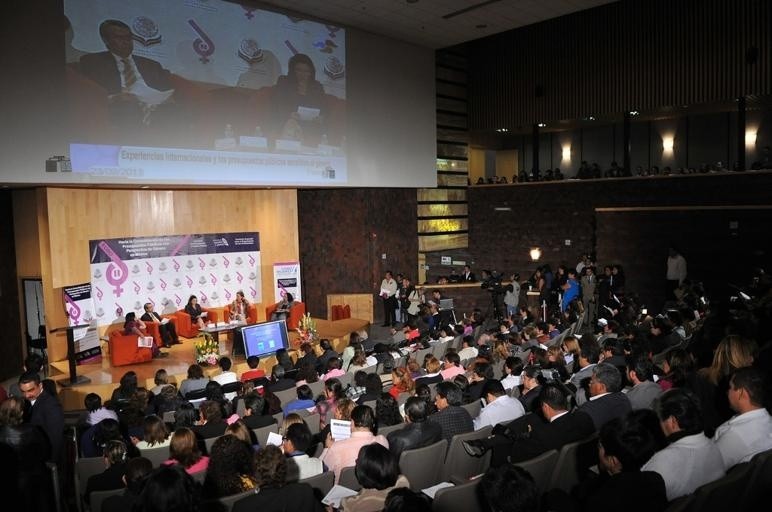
[0,301,772,512]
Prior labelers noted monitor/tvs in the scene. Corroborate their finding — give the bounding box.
[239,320,291,359]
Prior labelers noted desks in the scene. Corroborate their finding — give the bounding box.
[415,282,510,322]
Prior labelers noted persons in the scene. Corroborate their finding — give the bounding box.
[712,365,770,470]
[78,333,539,510]
[604,160,631,178]
[750,161,763,170]
[185,295,209,328]
[599,433,667,509]
[642,386,726,504]
[570,160,601,180]
[666,246,688,297]
[379,266,522,346]
[1,355,75,512]
[266,53,327,149]
[123,312,169,358]
[477,168,564,185]
[271,293,293,324]
[231,292,250,321]
[636,161,729,177]
[140,303,183,348]
[75,18,181,149]
[525,254,714,458]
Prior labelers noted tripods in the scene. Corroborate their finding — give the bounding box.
[491,294,503,327]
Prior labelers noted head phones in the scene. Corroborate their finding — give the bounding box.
[515,275,520,281]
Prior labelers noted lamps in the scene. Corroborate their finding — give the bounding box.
[530,248,542,264]
